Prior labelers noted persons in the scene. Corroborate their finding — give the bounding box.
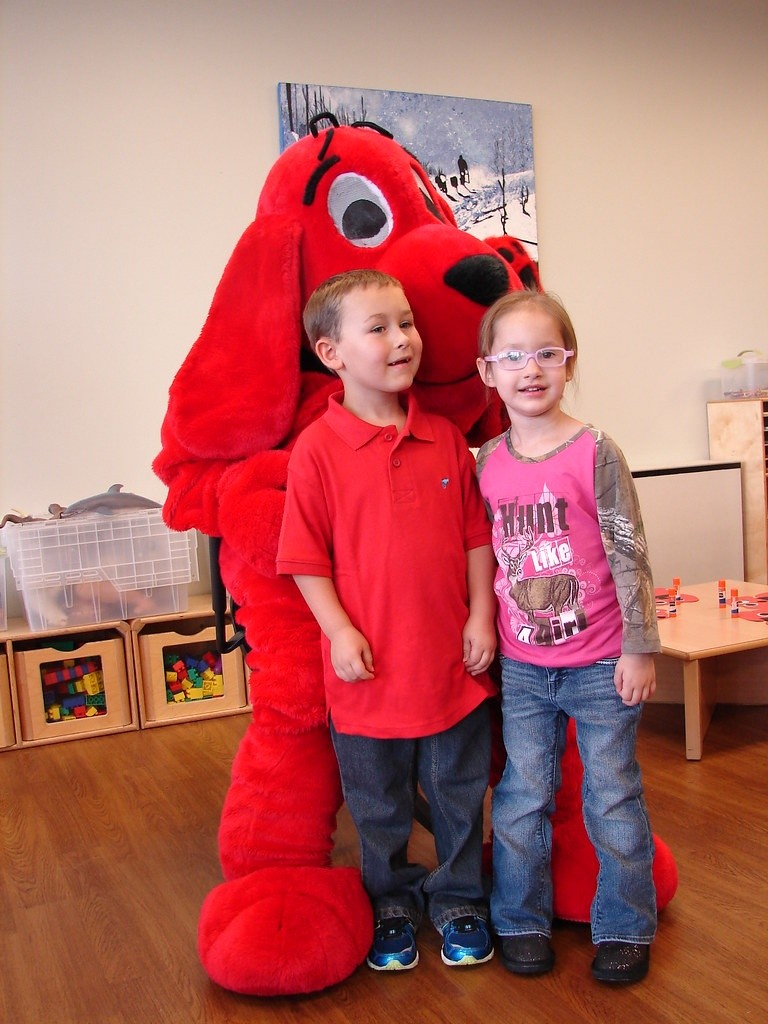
[456,154,468,187]
[275,270,496,972]
[475,288,665,986]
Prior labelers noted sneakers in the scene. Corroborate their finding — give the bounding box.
[591,940,649,983]
[440,916,495,967]
[500,933,555,975]
[367,915,421,971]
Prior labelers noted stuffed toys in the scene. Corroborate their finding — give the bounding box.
[152,112,680,994]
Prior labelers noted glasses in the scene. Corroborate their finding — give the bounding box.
[483,347,575,370]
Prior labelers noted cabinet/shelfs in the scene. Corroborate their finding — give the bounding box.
[707,398,768,585]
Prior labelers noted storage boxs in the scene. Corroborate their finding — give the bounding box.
[0,505,251,752]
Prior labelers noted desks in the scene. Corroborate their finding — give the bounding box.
[655,578,768,760]
